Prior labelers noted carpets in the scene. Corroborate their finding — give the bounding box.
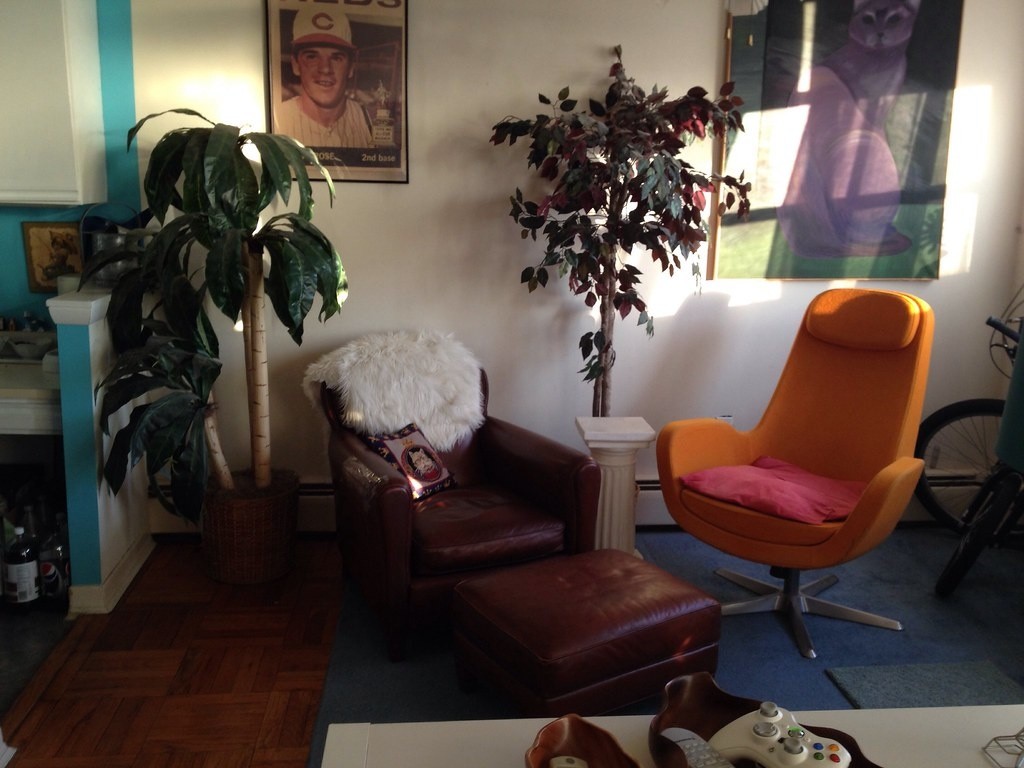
[307,525,1024,768]
[824,659,1024,709]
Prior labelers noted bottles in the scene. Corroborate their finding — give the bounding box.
[22,494,66,542]
[40,528,71,610]
[4,527,42,613]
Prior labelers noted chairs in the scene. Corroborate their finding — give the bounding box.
[655,289,935,658]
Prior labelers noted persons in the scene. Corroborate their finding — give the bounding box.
[281,7,377,149]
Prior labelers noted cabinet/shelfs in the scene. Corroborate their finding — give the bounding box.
[0,0,107,207]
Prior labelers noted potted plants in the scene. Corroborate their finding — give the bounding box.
[76,107,348,587]
[488,43,753,522]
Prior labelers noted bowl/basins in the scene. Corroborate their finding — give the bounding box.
[8,338,51,359]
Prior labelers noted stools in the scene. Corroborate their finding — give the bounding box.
[448,549,722,719]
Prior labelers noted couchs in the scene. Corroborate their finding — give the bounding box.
[301,326,601,665]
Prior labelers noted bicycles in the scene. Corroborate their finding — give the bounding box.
[915,287,1024,590]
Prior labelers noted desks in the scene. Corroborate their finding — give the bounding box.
[321,705,1024,768]
[575,416,656,562]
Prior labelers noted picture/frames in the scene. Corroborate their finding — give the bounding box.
[21,221,91,292]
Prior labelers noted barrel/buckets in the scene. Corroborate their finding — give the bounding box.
[79,200,143,289]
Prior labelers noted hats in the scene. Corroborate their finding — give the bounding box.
[288,3,357,48]
